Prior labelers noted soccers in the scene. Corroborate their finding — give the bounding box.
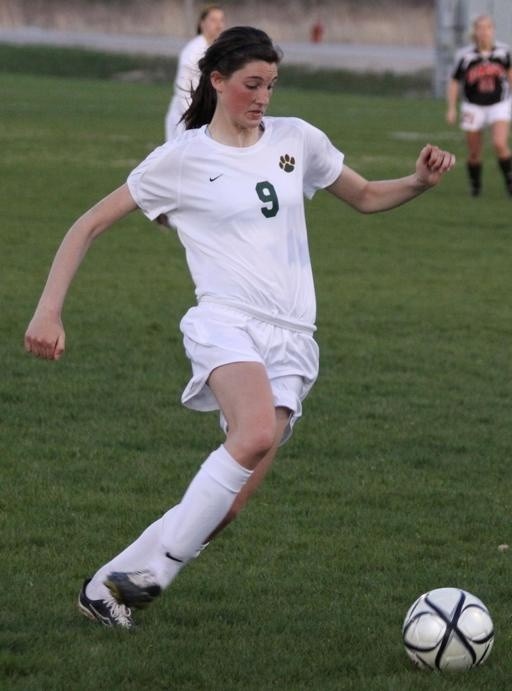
[401,587,493,672]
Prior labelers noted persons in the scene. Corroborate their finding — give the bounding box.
[163,5,228,143]
[445,12,512,203]
[20,24,457,634]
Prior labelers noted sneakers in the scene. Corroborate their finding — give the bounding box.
[103,569,161,610]
[78,578,138,636]
